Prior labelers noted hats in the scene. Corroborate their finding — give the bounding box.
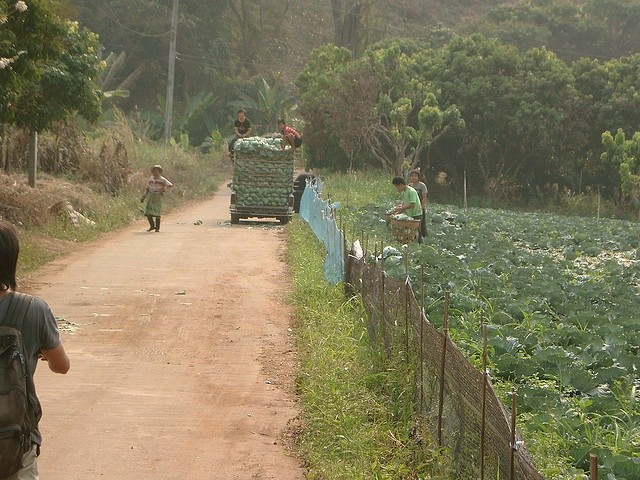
[150,165,163,174]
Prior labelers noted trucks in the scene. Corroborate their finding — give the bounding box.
[227,137,295,224]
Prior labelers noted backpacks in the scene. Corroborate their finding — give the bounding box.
[1,291,41,477]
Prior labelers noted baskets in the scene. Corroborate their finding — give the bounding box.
[390,216,420,244]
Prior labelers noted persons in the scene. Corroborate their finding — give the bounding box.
[227,109,251,163]
[277,119,303,151]
[140,165,173,232]
[0,218,70,480]
[392,177,425,244]
[408,171,428,237]
